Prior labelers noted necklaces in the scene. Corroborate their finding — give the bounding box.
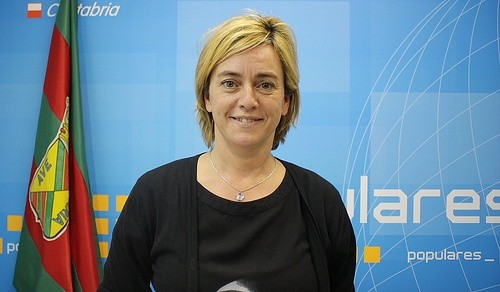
[209,146,277,201]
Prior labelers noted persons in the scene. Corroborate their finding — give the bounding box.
[95,10,356,292]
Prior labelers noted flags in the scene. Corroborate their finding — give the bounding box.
[13,1,104,292]
